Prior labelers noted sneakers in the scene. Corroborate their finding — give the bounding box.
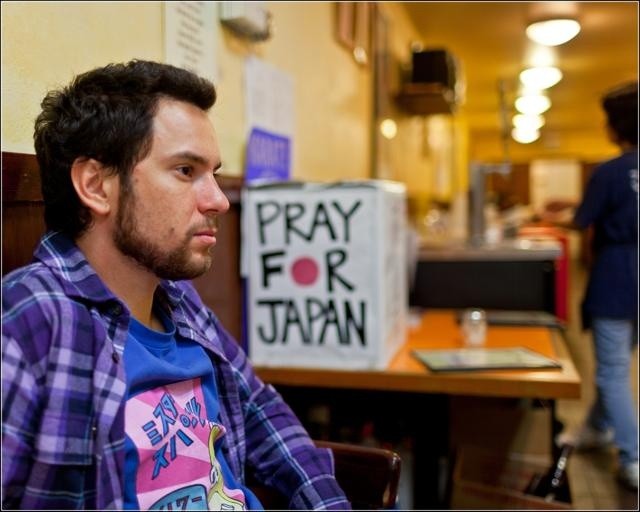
[558,427,609,451]
[612,461,640,490]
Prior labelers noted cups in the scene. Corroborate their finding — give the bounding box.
[460,309,488,354]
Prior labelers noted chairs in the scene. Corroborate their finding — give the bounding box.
[312,442,401,510]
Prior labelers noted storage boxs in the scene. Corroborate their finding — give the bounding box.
[452,440,575,511]
[240,177,408,373]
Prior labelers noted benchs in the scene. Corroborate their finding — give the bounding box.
[407,237,573,503]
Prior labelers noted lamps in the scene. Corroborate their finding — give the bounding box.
[515,1,583,114]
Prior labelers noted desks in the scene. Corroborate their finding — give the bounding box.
[248,310,582,510]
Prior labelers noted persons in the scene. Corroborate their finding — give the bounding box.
[552,77,639,504]
[1,56,354,511]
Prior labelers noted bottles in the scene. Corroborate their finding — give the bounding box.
[523,443,574,502]
